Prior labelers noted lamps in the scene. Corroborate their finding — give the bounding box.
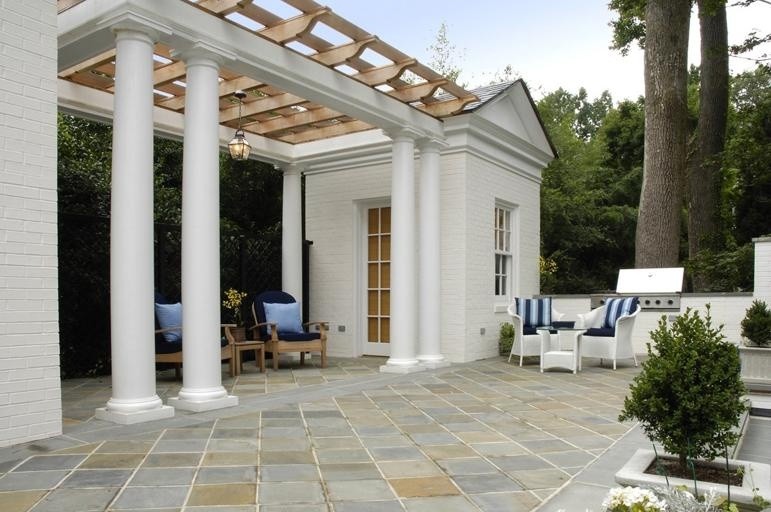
[226,91,252,163]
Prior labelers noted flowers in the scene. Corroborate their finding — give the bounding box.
[222,287,249,328]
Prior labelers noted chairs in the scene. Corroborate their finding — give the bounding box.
[248,291,331,372]
[506,299,565,367]
[153,290,237,384]
[574,297,642,374]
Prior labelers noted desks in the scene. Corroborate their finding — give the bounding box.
[528,321,596,373]
[228,339,265,376]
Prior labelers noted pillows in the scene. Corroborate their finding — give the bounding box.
[154,301,187,345]
[513,295,554,326]
[262,299,306,336]
[602,295,641,329]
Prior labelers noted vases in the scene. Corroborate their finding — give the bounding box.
[226,324,247,342]
